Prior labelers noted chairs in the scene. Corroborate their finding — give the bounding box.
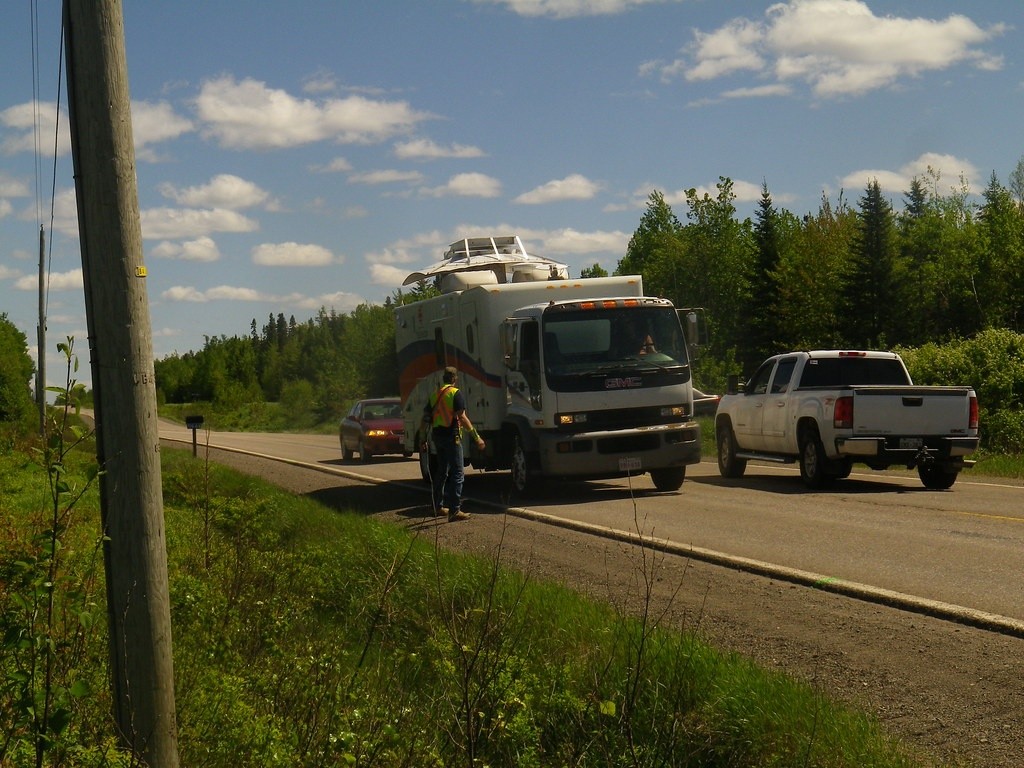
[364,412,374,418]
[534,331,572,365]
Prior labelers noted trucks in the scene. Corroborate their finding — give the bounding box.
[393,236,720,498]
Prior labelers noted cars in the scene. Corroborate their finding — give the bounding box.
[338,397,414,461]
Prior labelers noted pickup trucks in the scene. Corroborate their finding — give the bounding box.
[715,347,980,490]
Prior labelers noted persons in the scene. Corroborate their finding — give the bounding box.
[419,366,486,521]
[621,321,657,356]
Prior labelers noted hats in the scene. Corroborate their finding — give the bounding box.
[444,366,456,378]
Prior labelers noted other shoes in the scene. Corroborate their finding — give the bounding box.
[433,507,449,515]
[448,511,471,522]
